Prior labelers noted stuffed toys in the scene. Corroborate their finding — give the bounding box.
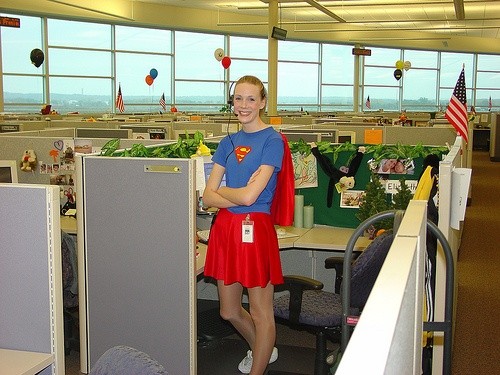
[19,149,38,172]
[311,142,366,208]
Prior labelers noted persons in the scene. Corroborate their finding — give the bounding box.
[202,75,293,375]
[374,158,412,174]
[38,164,77,205]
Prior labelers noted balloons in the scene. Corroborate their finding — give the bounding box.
[215,48,230,68]
[394,60,411,81]
[30,49,44,68]
[145,68,157,85]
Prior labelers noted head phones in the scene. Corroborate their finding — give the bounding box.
[225,81,237,106]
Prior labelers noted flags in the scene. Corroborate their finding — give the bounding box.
[116,85,125,113]
[488,98,492,110]
[366,96,371,109]
[445,68,469,143]
[159,93,166,110]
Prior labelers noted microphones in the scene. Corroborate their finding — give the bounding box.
[228,104,240,119]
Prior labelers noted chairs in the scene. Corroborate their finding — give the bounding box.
[60,228,80,356]
[274,229,394,375]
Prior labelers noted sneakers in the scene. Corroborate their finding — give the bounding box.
[238,346,278,373]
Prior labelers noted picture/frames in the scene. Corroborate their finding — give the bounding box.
[340,191,365,208]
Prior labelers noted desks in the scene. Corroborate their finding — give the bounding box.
[473,128,491,131]
[195,225,377,277]
